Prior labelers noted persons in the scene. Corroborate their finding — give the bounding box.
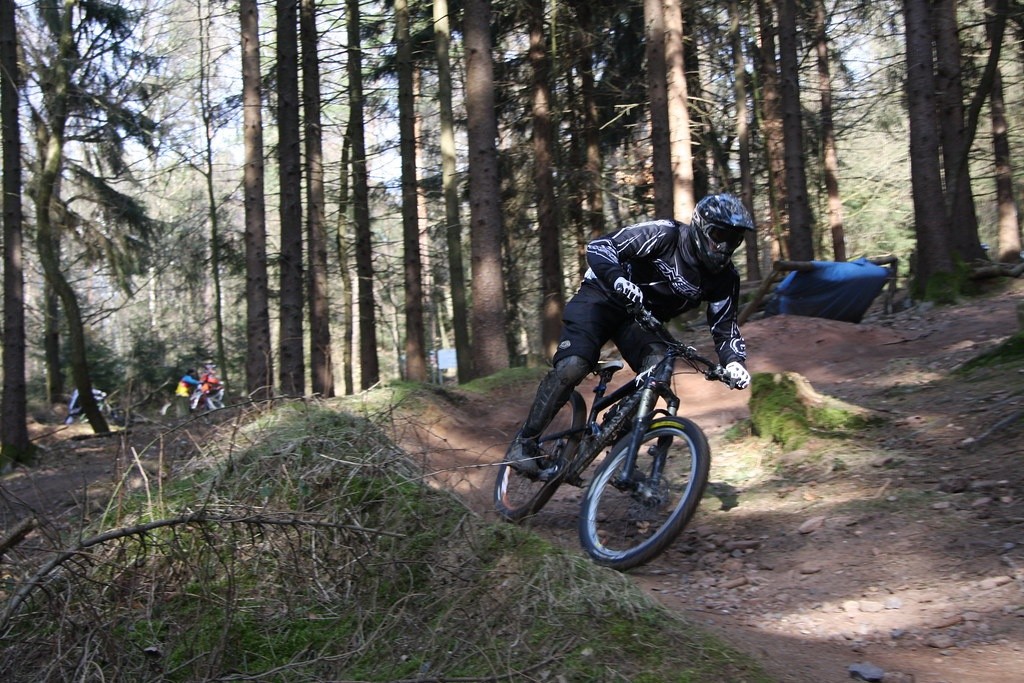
[506,193,753,479]
[174,369,224,410]
[65,388,107,425]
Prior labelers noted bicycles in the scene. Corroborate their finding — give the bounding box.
[160,384,224,423]
[491,300,741,576]
[63,399,111,427]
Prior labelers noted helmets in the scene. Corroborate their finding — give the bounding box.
[689,193,754,270]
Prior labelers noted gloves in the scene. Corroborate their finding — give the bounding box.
[612,276,643,304]
[718,360,751,390]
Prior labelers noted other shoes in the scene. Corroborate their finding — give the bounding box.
[595,451,645,481]
[505,428,539,475]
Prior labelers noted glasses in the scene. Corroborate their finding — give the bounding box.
[705,223,745,254]
